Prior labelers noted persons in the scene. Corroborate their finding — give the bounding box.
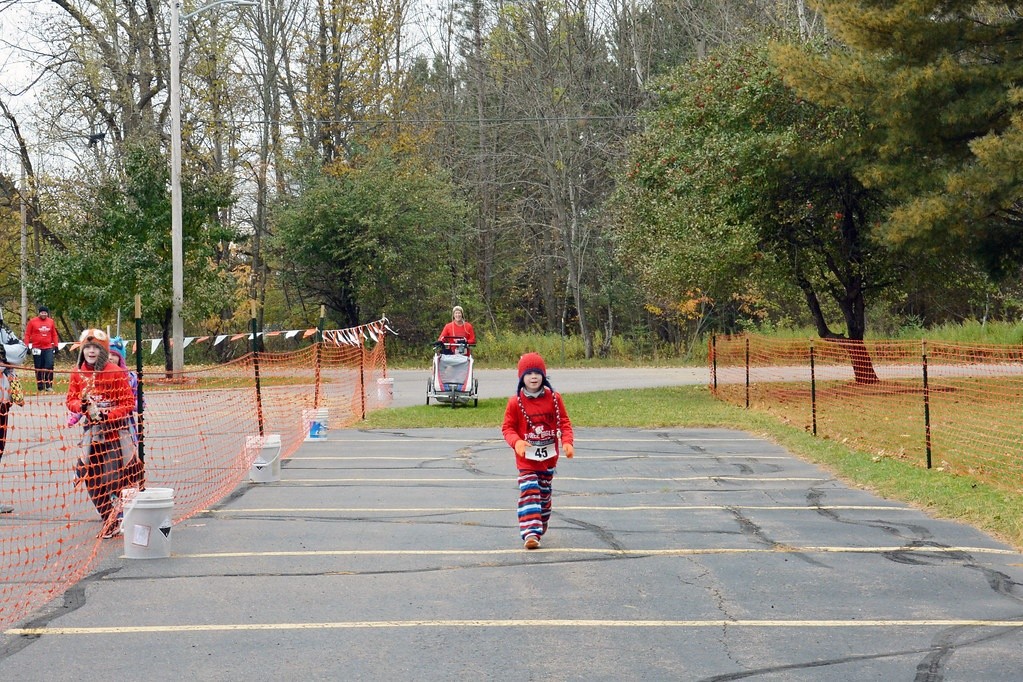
[0,307,28,512]
[501,353,574,549]
[24,306,58,395]
[438,306,475,390]
[65,328,146,537]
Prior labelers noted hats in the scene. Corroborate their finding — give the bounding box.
[517,353,561,438]
[109,335,128,373]
[452,306,470,337]
[77,329,109,400]
[38,306,49,313]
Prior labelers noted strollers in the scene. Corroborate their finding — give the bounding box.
[425,335,479,409]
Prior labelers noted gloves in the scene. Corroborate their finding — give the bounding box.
[27,348,31,355]
[54,347,60,357]
[515,440,532,457]
[563,443,574,459]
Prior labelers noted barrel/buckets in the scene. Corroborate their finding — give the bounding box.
[377,378,393,401]
[247,435,281,483]
[121,489,174,559]
[302,408,327,441]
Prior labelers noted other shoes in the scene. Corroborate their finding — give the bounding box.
[102,520,120,538]
[38,390,44,392]
[46,387,54,392]
[0,504,14,513]
[524,534,540,549]
